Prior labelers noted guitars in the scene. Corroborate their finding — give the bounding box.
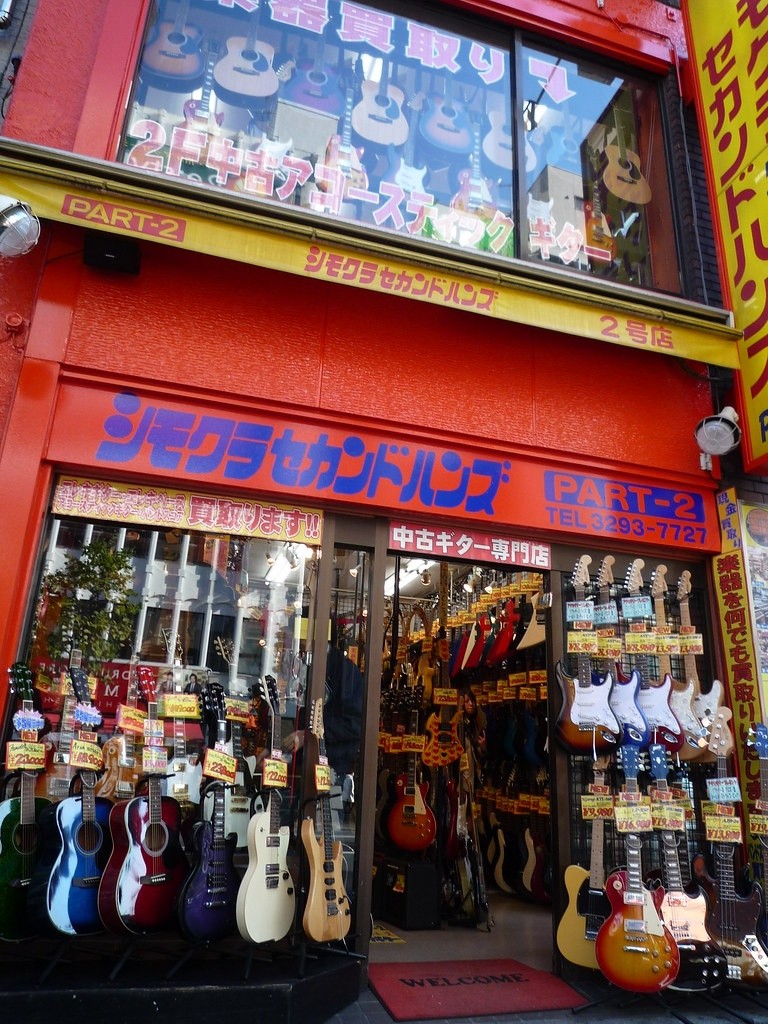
[686,705,768,991]
[675,569,736,763]
[138,1,652,265]
[42,664,113,939]
[590,555,651,752]
[234,673,298,946]
[380,594,495,929]
[615,558,686,754]
[96,665,193,943]
[175,680,244,946]
[0,662,58,945]
[446,565,556,904]
[646,744,729,991]
[554,755,612,971]
[593,743,681,994]
[648,563,708,761]
[292,696,355,944]
[552,552,625,754]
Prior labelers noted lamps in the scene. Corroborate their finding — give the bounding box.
[484,569,496,593]
[464,565,482,592]
[286,550,299,570]
[0,195,41,257]
[694,406,742,472]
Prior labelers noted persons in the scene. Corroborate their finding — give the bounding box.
[161,671,201,695]
[280,642,366,884]
[449,691,488,795]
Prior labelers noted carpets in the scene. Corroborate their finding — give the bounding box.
[369,959,590,1022]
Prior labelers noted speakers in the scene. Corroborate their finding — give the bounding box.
[83,233,141,276]
